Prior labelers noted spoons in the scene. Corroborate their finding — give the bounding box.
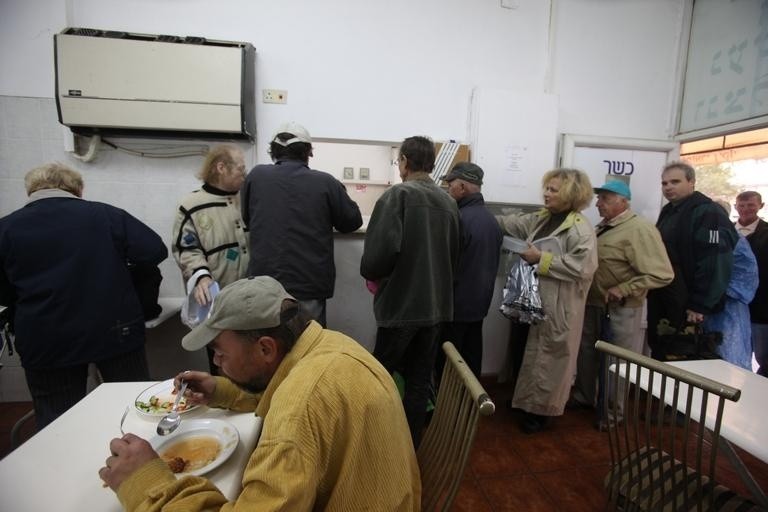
[156,378,189,437]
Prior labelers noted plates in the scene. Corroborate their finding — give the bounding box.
[143,419,242,475]
[134,376,205,417]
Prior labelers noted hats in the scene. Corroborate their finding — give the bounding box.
[593,179,631,198]
[439,162,483,184]
[269,125,311,147]
[181,275,296,352]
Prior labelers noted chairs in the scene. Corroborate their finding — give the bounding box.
[595,340,768,512]
[416,341,495,512]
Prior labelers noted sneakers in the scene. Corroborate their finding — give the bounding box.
[571,390,597,408]
[601,411,623,430]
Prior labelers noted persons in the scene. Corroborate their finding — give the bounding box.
[564,177,676,430]
[92,274,427,512]
[441,161,498,382]
[0,162,168,425]
[358,134,461,452]
[732,190,768,375]
[710,201,759,374]
[490,169,599,433]
[239,123,364,329]
[639,159,738,432]
[175,143,252,377]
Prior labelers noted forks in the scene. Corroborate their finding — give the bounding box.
[102,406,131,488]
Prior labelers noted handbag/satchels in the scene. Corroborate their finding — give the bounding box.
[500,257,545,325]
